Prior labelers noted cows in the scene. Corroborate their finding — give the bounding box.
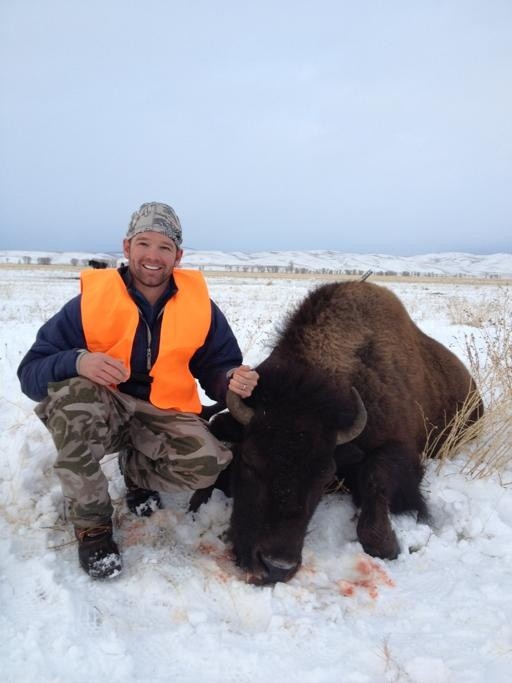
[187,279,485,586]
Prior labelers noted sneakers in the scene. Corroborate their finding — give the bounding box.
[118,452,162,517]
[76,519,120,579]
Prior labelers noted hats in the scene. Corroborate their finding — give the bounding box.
[126,202,183,247]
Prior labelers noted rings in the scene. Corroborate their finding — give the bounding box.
[241,383,247,390]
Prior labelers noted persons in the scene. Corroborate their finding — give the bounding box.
[13,201,261,582]
